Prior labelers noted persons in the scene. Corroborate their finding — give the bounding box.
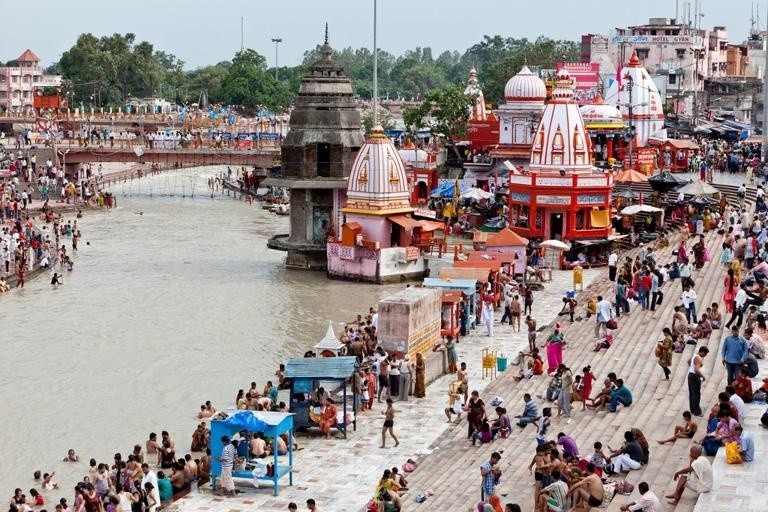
[237,166,291,214]
[432,237,634,448]
[0,126,285,152]
[429,135,526,234]
[227,166,232,181]
[191,305,426,511]
[665,127,768,184]
[528,429,662,511]
[665,446,713,505]
[0,149,117,291]
[634,184,768,311]
[655,302,768,463]
[1,428,195,511]
[472,452,522,511]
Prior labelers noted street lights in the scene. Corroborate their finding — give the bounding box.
[686,3,693,30]
[697,9,706,30]
[747,10,756,33]
[623,75,648,169]
[57,148,70,178]
[271,35,282,83]
[414,115,436,169]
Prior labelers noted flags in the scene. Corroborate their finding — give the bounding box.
[616,58,622,85]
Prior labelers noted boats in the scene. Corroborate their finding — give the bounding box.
[261,201,292,215]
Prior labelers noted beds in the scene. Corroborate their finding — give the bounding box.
[206,409,297,496]
[658,139,700,173]
[283,355,360,439]
[421,251,518,343]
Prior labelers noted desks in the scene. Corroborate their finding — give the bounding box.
[467,213,484,227]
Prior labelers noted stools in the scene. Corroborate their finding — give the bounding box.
[480,348,497,381]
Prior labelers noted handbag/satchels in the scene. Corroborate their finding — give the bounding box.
[725,441,743,464]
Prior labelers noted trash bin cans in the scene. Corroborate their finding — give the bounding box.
[496,356,508,371]
